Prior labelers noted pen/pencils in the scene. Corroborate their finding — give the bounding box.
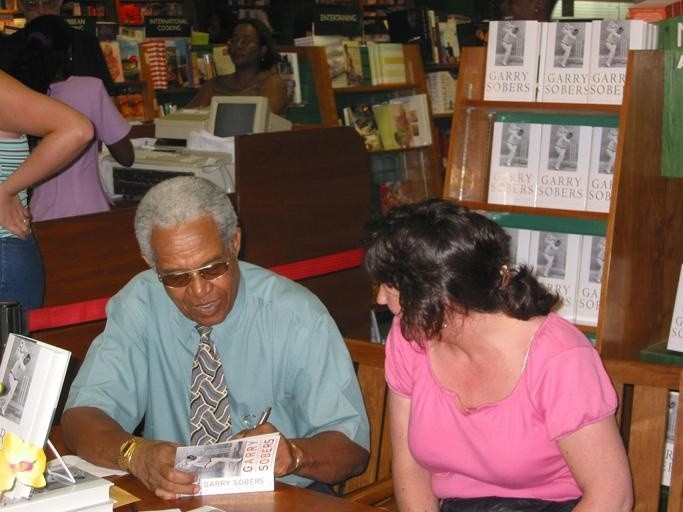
[258,407,271,426]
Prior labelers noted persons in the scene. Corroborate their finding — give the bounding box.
[362,199,634,511]
[0,13,133,359]
[59,176,372,502]
[180,449,246,473]
[184,18,288,121]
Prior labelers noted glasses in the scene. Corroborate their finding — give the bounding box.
[155,245,232,289]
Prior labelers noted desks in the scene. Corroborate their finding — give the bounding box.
[1,422,385,511]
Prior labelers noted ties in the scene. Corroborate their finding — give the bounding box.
[191,324,232,447]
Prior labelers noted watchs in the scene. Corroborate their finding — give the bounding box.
[116,436,143,472]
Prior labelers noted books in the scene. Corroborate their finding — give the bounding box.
[0,332,143,512]
[298,5,475,148]
[487,121,619,213]
[169,432,282,500]
[501,226,606,326]
[138,33,238,89]
[483,21,658,105]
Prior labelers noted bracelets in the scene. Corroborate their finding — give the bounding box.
[287,439,305,474]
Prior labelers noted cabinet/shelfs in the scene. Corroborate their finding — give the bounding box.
[278,41,442,208]
[442,46,682,360]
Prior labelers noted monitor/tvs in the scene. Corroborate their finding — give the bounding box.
[207,96,293,138]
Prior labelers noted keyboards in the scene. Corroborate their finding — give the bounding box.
[102,146,231,172]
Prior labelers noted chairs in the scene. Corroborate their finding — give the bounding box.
[331,337,389,495]
[345,361,682,511]
